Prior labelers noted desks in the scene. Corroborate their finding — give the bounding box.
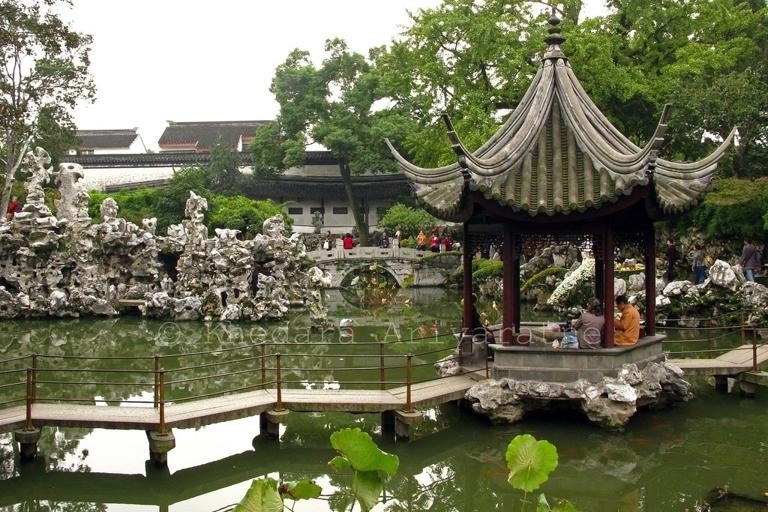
[531,325,575,347]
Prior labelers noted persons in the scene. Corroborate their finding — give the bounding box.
[331,315,452,341]
[532,294,642,348]
[462,294,496,343]
[6,196,22,224]
[319,229,454,258]
[691,243,706,284]
[737,237,761,283]
[664,240,680,281]
